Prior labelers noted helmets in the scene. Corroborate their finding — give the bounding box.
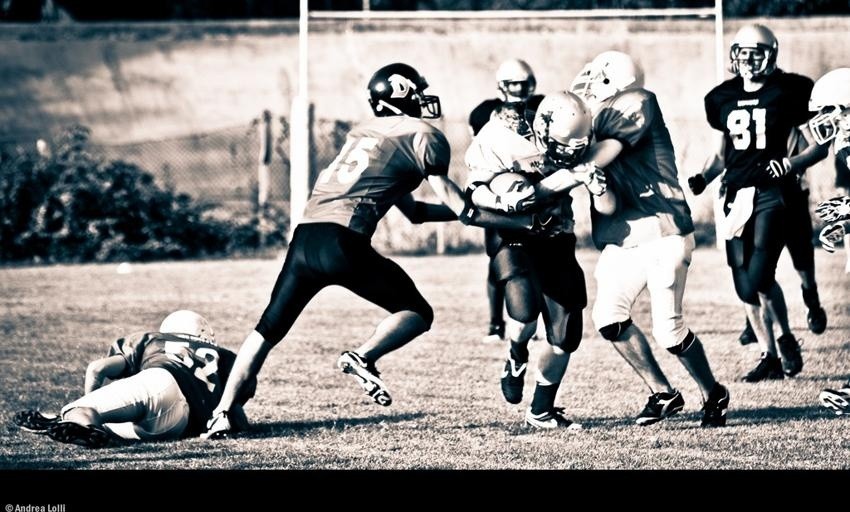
[159,309,215,342]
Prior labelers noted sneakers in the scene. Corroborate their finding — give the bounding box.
[199,410,232,439]
[12,409,111,449]
[336,350,392,407]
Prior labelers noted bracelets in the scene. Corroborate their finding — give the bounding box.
[458,200,477,226]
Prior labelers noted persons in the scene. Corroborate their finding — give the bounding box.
[14,310,257,448]
[463,22,849,428]
[203,62,565,440]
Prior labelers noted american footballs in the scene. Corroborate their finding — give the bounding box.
[489,173,532,194]
